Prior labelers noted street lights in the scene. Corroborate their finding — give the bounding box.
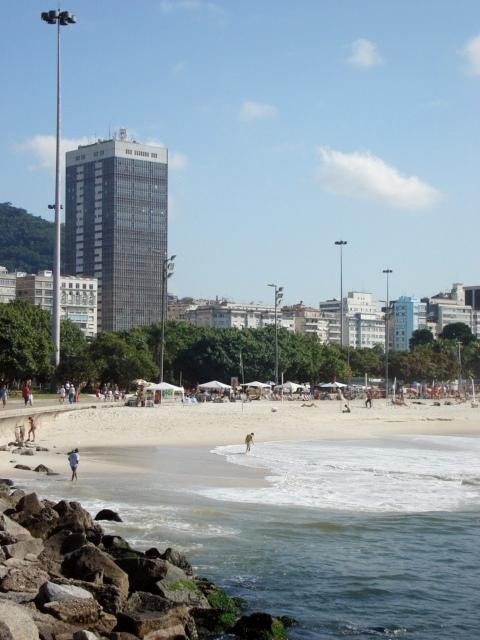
[265,283,284,385]
[154,249,176,384]
[39,9,76,394]
[381,269,392,393]
[334,241,347,352]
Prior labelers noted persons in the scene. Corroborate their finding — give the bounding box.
[68,448,81,480]
[57,381,79,404]
[365,392,372,408]
[343,404,350,413]
[22,383,33,406]
[27,416,36,442]
[96,383,120,397]
[245,432,254,452]
[0,384,8,408]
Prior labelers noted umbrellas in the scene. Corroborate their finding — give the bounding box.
[317,382,347,393]
